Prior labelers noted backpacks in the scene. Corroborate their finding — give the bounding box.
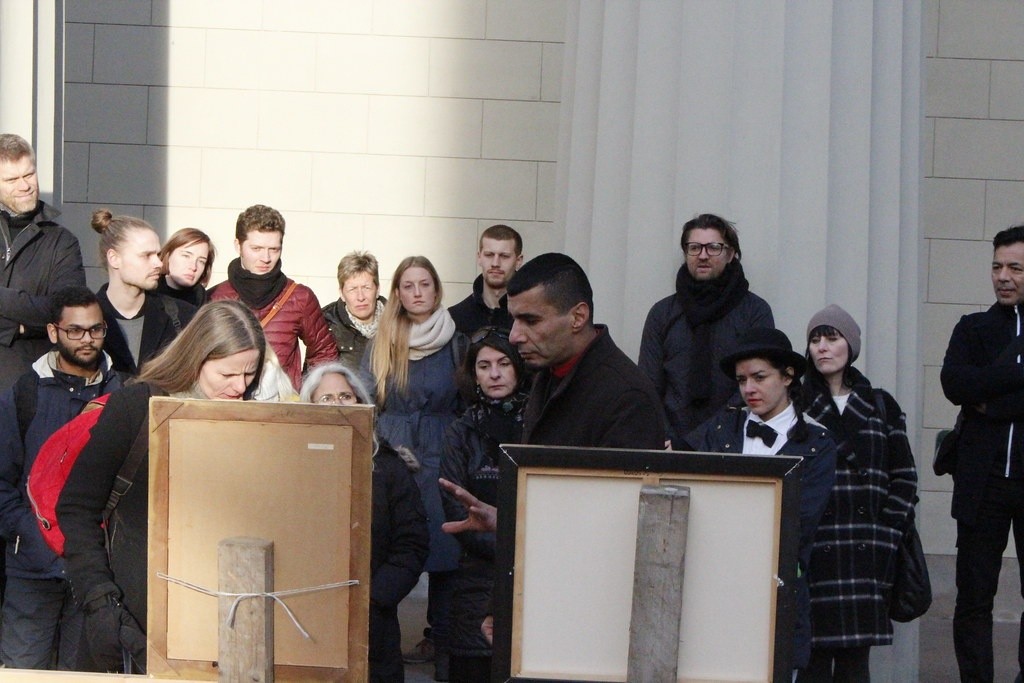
[25,382,163,557]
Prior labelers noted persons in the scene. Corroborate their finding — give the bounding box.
[440,254,665,683]
[206,205,338,395]
[358,256,476,683]
[939,225,1024,683]
[0,134,85,394]
[148,228,215,334]
[696,328,838,683]
[638,213,774,452]
[301,251,388,403]
[0,285,135,671]
[54,299,266,675]
[300,361,427,683]
[401,225,523,664]
[441,327,528,682]
[91,209,178,375]
[794,305,919,683]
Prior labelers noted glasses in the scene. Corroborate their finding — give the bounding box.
[52,321,108,340]
[470,326,512,344]
[684,240,731,256]
[313,395,358,405]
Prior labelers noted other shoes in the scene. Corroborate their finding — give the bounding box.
[402,641,436,663]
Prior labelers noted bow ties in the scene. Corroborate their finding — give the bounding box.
[746,421,778,447]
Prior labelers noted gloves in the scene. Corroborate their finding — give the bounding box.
[82,583,143,671]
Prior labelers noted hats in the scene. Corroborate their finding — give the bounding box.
[805,304,861,364]
[727,328,807,375]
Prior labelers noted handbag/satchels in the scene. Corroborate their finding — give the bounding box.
[890,524,932,622]
